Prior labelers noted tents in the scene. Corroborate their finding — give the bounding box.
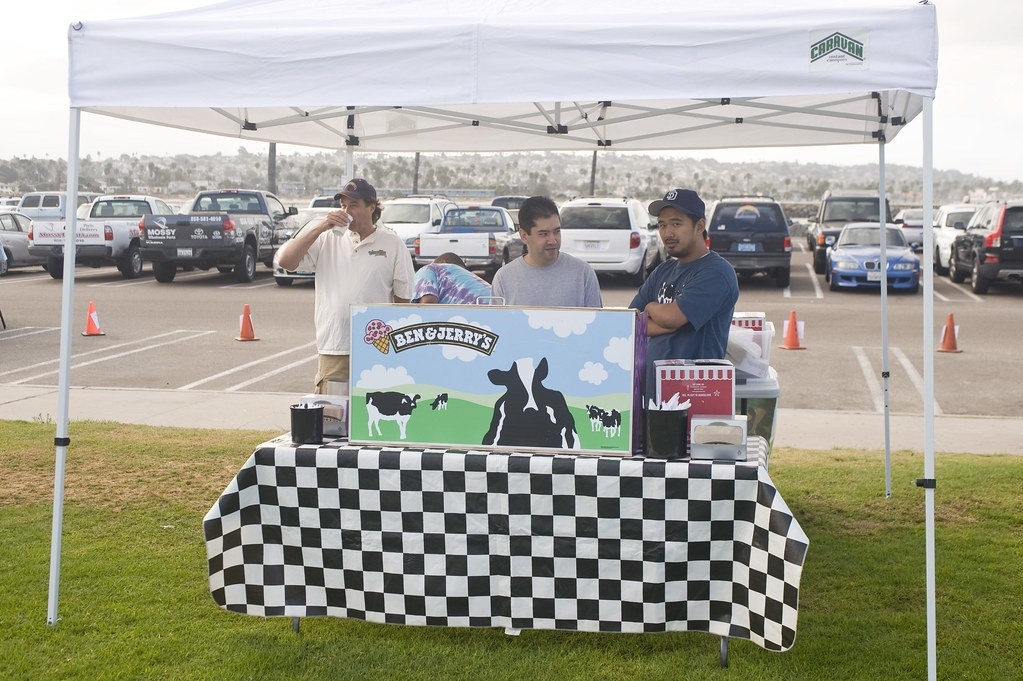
[42,1,944,681]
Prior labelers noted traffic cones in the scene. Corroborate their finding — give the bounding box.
[937,313,964,353]
[776,310,808,350]
[81,301,106,336]
[235,304,260,342]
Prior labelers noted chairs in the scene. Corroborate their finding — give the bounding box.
[208,203,221,211]
[247,202,259,211]
[720,212,737,222]
[483,217,498,225]
[101,205,115,215]
[454,217,469,225]
[754,212,773,224]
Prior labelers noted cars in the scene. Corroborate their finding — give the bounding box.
[931,204,982,276]
[826,222,921,295]
[164,196,236,215]
[76,201,134,219]
[0,211,48,277]
[0,196,22,210]
[892,208,939,246]
[378,194,456,256]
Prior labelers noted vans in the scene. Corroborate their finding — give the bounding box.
[15,192,105,219]
[492,195,530,232]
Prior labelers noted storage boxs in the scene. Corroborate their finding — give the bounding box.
[731,311,765,357]
[691,414,748,461]
[651,360,736,455]
[296,393,349,438]
[726,322,773,379]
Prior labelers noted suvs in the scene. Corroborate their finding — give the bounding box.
[558,196,667,288]
[947,199,1023,295]
[703,195,795,289]
[807,189,904,275]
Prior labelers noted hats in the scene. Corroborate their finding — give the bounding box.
[334,178,377,200]
[649,189,705,219]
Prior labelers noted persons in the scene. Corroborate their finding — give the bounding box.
[409,252,493,305]
[490,196,604,307]
[277,178,417,396]
[627,189,738,414]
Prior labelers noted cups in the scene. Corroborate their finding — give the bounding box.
[332,213,353,237]
[644,405,688,460]
[289,404,324,445]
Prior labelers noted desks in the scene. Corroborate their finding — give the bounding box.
[736,366,780,442]
[202,430,811,670]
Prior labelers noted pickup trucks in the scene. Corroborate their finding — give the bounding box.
[414,205,528,285]
[295,196,342,227]
[138,188,303,284]
[27,195,175,280]
